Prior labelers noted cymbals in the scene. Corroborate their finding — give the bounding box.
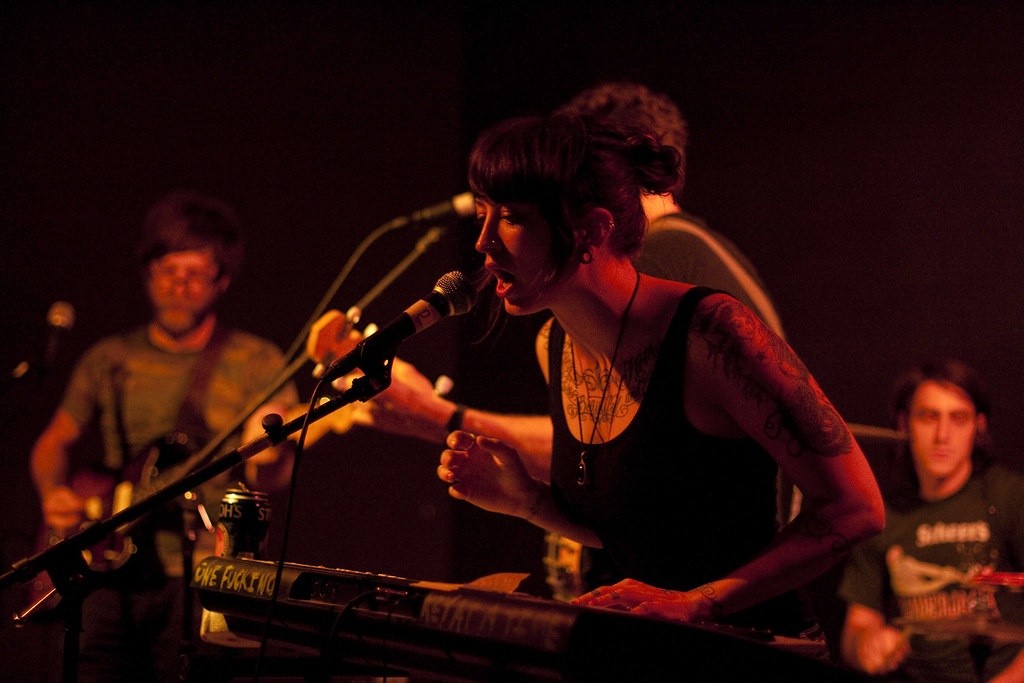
[846,422,907,441]
[902,616,1024,643]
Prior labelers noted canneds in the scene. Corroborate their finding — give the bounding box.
[213,481,272,560]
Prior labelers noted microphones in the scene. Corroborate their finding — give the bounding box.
[42,300,75,387]
[320,271,479,383]
[396,192,476,230]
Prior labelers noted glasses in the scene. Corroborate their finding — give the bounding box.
[151,265,224,295]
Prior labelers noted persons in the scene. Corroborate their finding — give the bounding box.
[436,115,887,625]
[828,360,1023,683]
[30,192,299,580]
[331,85,801,528]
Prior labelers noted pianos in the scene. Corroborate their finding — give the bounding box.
[186,549,870,683]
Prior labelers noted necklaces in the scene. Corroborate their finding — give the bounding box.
[571,271,641,486]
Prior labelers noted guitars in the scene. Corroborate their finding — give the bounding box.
[302,305,591,598]
[19,463,270,621]
